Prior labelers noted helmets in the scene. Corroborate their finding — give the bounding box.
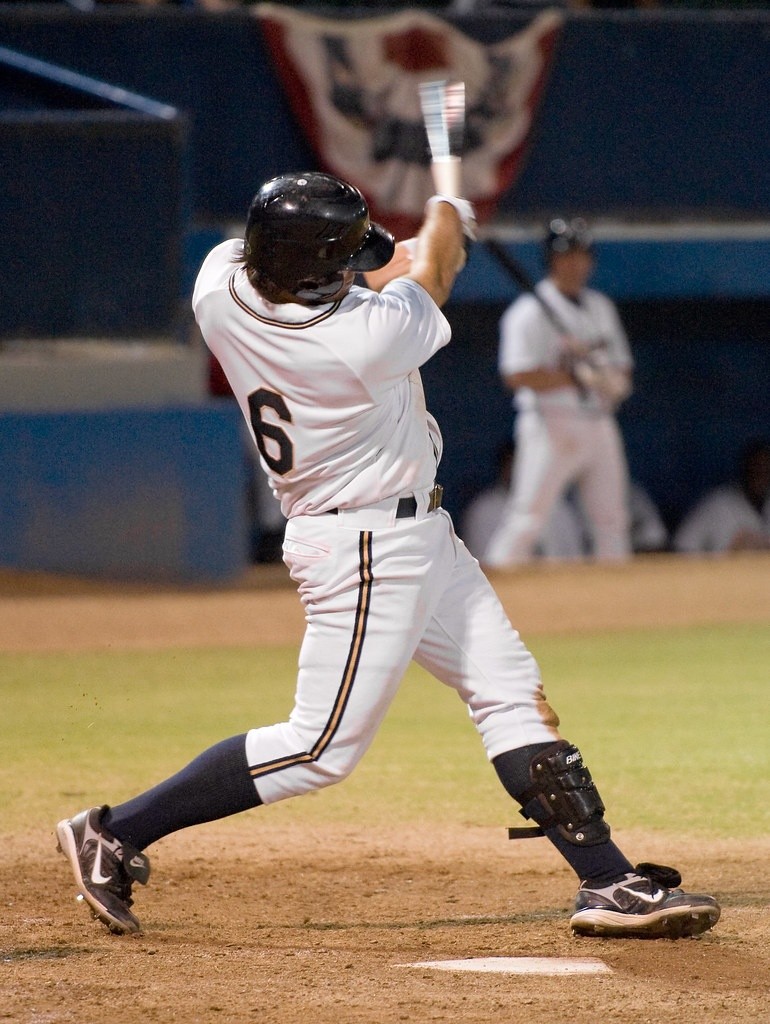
[538,215,601,256]
[244,167,398,303]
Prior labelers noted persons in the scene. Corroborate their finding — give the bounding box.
[195,183,770,562]
[60,169,727,941]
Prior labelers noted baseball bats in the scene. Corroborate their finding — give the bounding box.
[476,235,596,358]
[419,78,467,207]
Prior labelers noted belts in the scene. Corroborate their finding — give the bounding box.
[276,484,455,518]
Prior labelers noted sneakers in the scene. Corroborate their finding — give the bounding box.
[58,805,147,934]
[572,873,724,943]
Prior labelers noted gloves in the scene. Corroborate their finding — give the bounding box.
[424,192,471,239]
